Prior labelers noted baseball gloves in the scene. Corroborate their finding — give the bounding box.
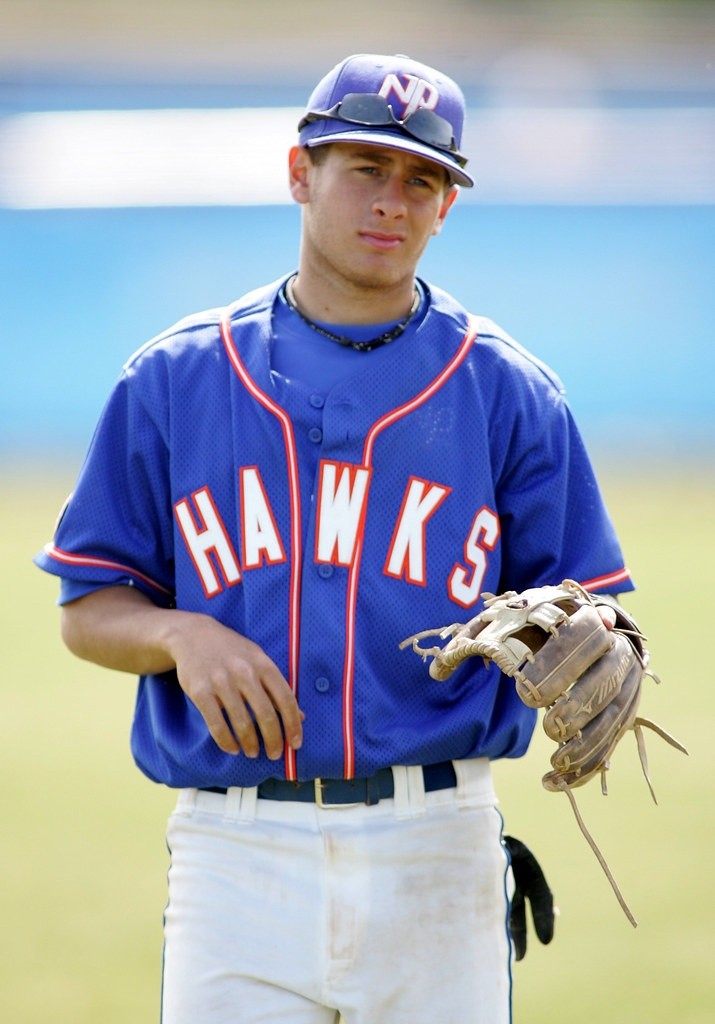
[422,583,645,794]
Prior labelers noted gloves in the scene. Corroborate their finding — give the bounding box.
[503,834,555,962]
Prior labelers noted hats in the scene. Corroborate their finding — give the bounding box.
[298,55,474,188]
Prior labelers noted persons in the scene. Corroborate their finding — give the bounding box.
[34,52,688,1024]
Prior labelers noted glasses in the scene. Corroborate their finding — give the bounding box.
[298,93,468,170]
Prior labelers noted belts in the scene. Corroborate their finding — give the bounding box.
[196,759,457,812]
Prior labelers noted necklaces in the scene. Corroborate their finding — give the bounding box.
[285,275,420,353]
